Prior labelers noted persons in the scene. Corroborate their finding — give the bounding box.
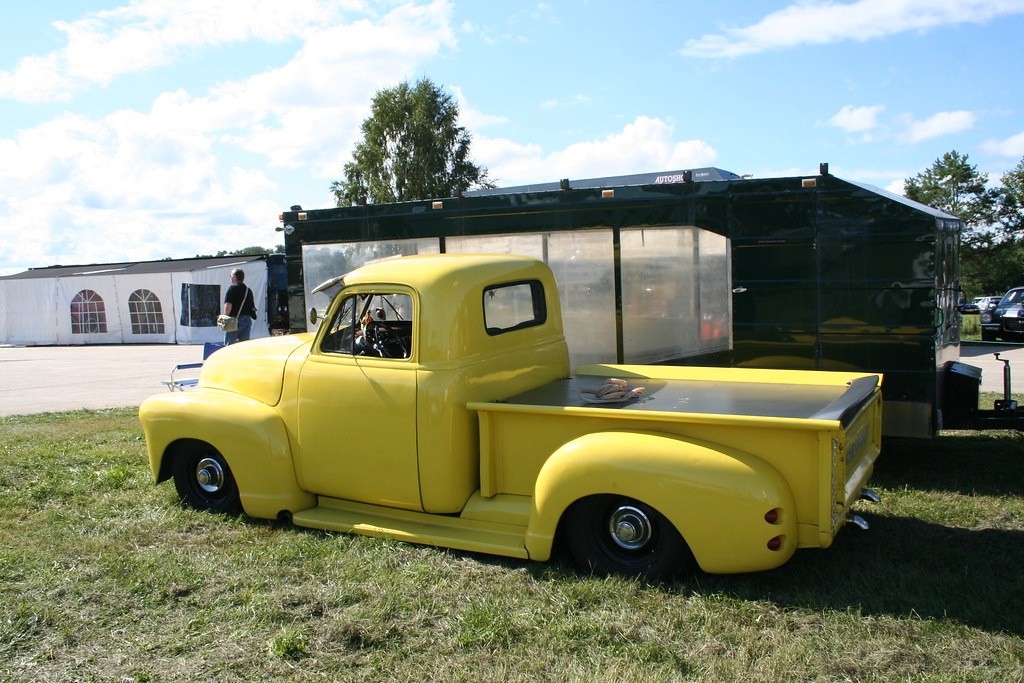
[360,309,376,331]
[224,268,257,346]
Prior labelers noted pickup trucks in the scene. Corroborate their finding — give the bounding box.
[137,252,884,584]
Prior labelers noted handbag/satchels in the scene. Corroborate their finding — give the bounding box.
[217,315,239,333]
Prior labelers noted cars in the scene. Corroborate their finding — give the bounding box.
[957,287,1024,342]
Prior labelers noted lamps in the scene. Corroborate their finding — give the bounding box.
[359,196,365,205]
[298,213,308,221]
[289,205,303,210]
[801,179,818,186]
[559,179,574,190]
[433,202,443,209]
[820,162,830,175]
[276,227,285,231]
[452,187,464,197]
[683,170,693,183]
[601,190,614,199]
